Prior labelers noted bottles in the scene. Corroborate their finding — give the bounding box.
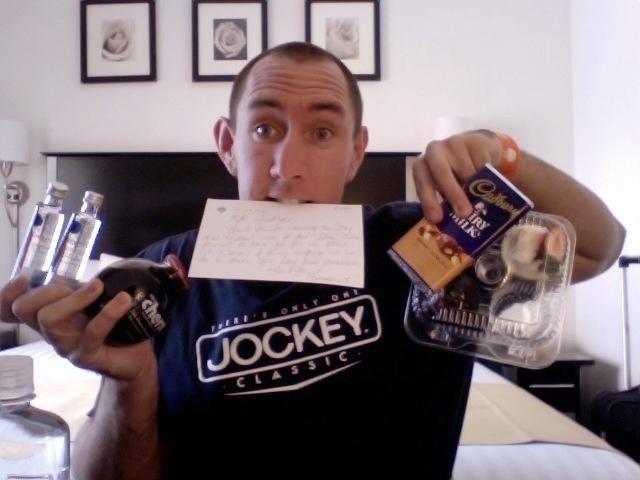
[11,181,103,292]
[83,255,191,346]
[1,354,70,480]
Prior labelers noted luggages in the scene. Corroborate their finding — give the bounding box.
[587,383,640,464]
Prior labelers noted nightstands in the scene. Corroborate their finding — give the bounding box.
[503,347,594,420]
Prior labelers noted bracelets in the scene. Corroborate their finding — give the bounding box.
[496,130,522,182]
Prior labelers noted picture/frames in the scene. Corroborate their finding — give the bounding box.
[80,0,158,82]
[192,2,268,81]
[305,0,381,81]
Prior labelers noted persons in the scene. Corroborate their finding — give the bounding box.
[0,41,627,480]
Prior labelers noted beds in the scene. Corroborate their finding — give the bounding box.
[1,147,638,480]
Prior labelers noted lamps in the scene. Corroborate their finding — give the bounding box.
[1,119,33,228]
